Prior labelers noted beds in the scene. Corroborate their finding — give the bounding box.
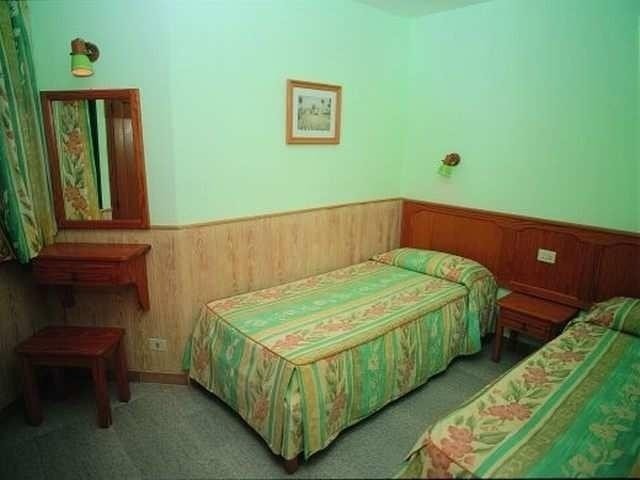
[182,248,499,475]
[392,294,639,480]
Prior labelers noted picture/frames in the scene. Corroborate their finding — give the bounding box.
[287,79,341,143]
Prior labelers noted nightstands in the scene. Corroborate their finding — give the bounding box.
[493,292,577,362]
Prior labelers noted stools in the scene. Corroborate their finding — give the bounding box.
[15,326,130,428]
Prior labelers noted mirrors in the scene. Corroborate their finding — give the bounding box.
[40,89,150,230]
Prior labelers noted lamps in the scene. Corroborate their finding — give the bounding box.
[69,38,99,76]
[438,153,460,176]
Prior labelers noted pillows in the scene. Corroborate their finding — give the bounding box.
[585,296,640,336]
[371,247,496,298]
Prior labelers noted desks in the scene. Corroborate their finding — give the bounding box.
[33,242,152,310]
